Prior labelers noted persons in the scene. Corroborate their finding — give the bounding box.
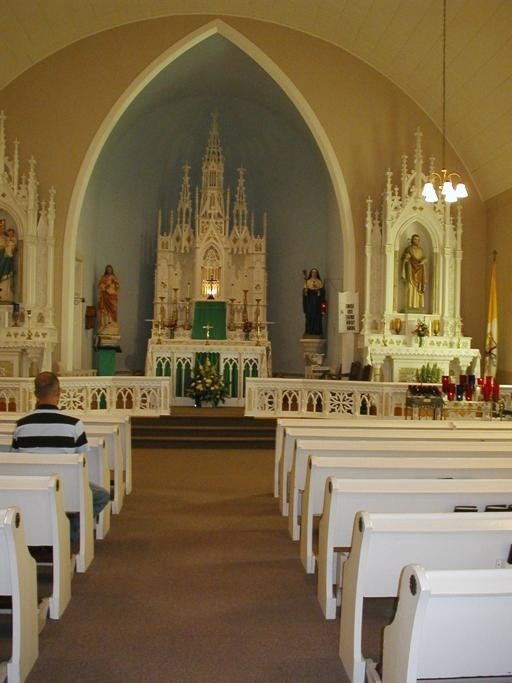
[97,263,122,335]
[11,371,111,561]
[302,267,327,334]
[401,232,428,311]
[0,219,17,284]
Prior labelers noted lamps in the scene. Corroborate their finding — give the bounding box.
[419,0,469,205]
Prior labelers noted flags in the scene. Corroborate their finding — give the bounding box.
[483,259,500,386]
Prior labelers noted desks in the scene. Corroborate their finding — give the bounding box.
[441,391,505,421]
[404,389,444,419]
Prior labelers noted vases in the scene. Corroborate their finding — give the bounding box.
[199,400,214,408]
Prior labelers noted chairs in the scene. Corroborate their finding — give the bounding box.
[331,361,373,413]
[322,359,361,379]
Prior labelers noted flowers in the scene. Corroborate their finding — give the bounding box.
[190,354,229,409]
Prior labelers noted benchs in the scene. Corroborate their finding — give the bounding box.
[269,412,511,683]
[0,402,133,682]
